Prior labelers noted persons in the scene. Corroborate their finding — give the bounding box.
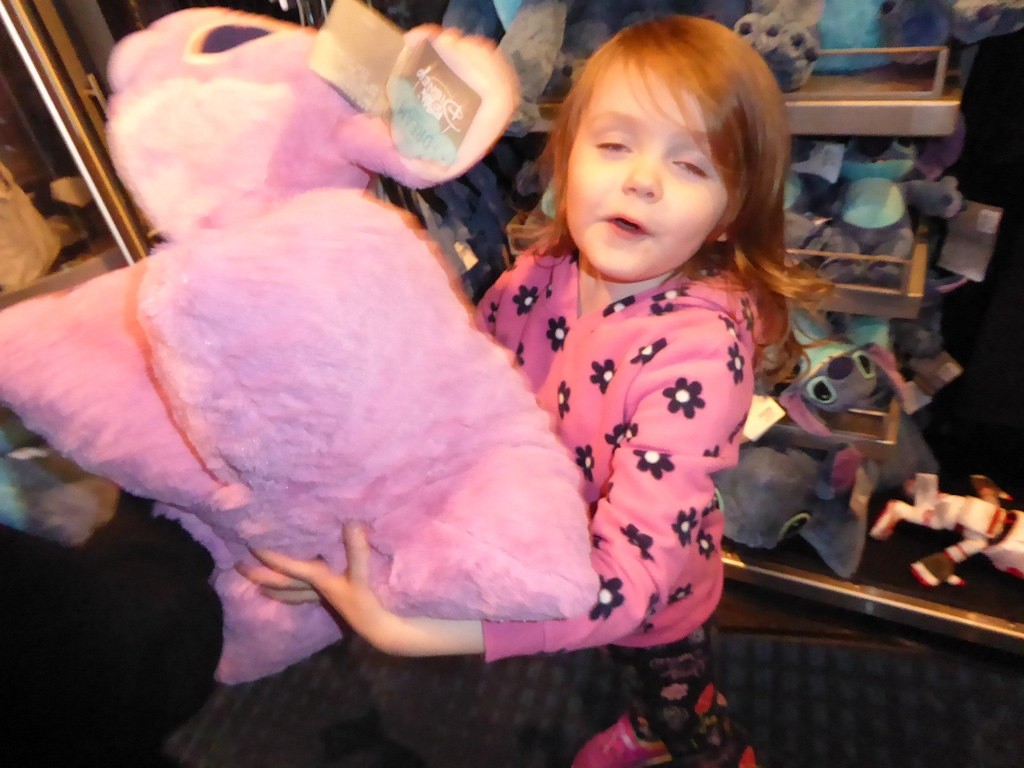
[236,13,845,768]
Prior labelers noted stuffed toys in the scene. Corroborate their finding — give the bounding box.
[441,0,824,138]
[0,0,601,684]
[712,0,1024,588]
[0,447,223,768]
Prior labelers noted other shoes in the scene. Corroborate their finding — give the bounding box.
[570,713,671,768]
[739,744,756,768]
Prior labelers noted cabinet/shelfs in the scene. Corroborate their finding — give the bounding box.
[507,44,1024,654]
